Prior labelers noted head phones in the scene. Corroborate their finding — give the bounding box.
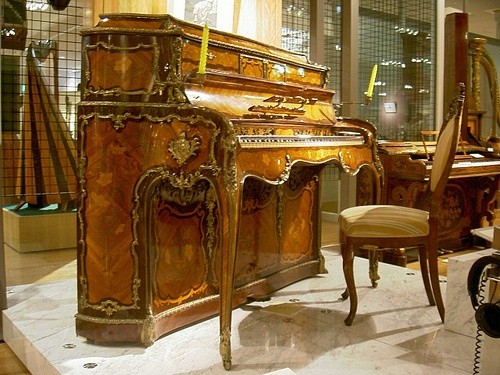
[467,254,500,338]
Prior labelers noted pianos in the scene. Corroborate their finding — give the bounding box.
[353,130,500,268]
[75,14,384,371]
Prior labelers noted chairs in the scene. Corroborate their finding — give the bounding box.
[337,83,466,326]
